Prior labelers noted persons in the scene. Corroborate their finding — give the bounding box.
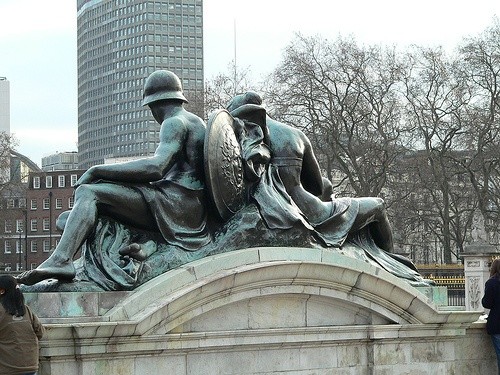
[226,90,395,255]
[11,69,213,285]
[481,259,500,375]
[0,274,45,375]
